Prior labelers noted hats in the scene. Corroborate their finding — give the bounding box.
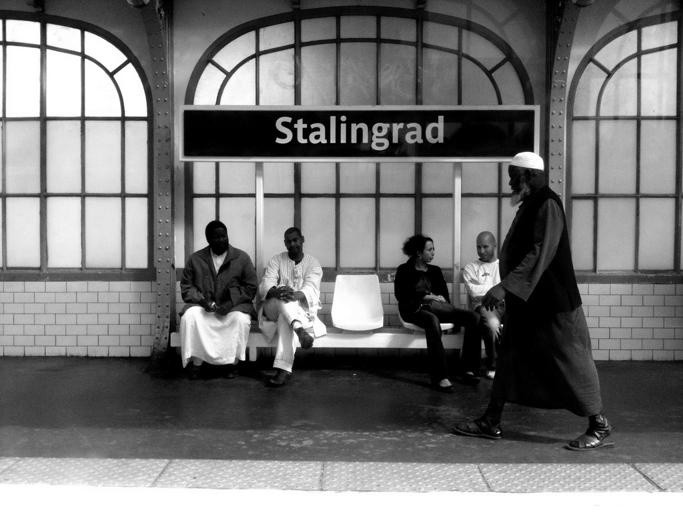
[508,150,545,171]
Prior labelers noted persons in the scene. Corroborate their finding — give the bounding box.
[390,232,491,394]
[449,146,621,452]
[178,219,259,380]
[254,227,327,388]
[458,230,502,381]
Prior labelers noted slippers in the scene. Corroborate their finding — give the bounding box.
[567,433,617,452]
[454,416,504,439]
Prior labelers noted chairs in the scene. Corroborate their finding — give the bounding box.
[169,274,502,362]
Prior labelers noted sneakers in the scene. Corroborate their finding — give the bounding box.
[270,369,293,386]
[197,365,236,379]
[431,367,497,387]
[301,333,313,349]
